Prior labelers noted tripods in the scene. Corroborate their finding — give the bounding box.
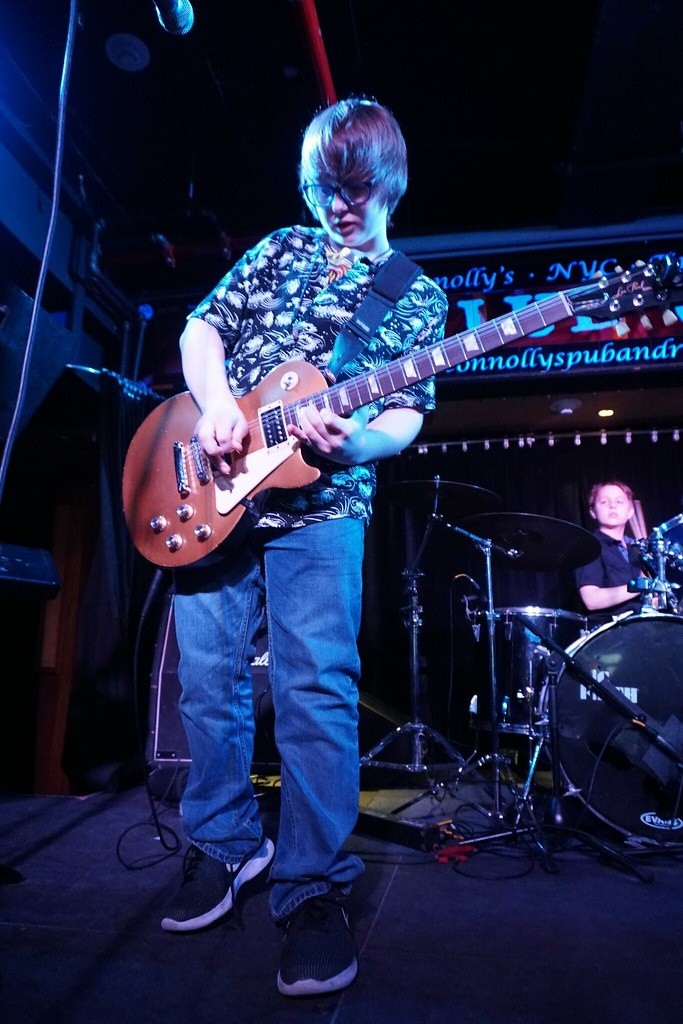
[390,517,654,882]
[350,499,510,808]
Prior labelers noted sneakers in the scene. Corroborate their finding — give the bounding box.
[160,833,275,931]
[276,884,357,996]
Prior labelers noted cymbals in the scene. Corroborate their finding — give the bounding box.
[372,478,504,525]
[441,510,603,571]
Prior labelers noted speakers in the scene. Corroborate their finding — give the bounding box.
[145,586,285,774]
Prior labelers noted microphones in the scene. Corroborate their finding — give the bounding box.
[148,0,193,37]
[467,575,490,606]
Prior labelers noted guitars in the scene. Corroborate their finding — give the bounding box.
[120,252,683,572]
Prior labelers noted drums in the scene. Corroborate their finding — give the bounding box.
[467,605,587,742]
[542,608,683,855]
[639,512,683,596]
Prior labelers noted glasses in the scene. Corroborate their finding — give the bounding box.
[303,173,379,208]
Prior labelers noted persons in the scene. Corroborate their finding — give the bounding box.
[575,481,662,632]
[158,92,448,997]
